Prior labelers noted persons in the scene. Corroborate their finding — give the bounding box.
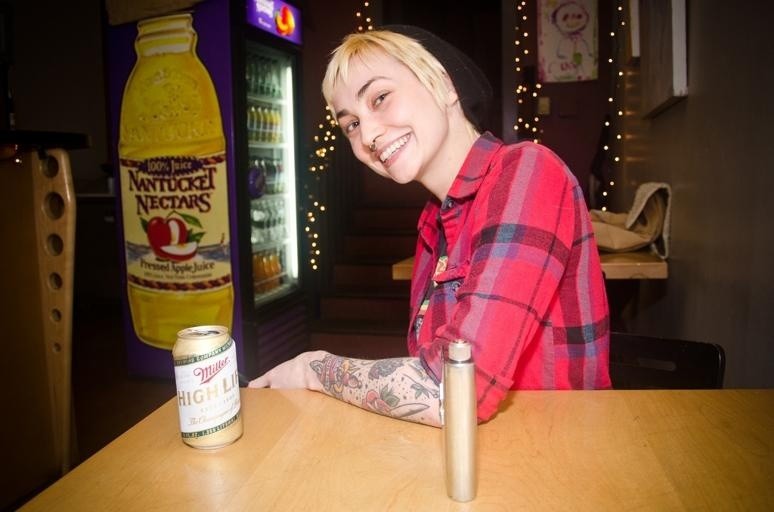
[245,31,615,429]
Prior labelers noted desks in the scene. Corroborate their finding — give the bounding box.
[13,387,774,511]
[390,256,671,282]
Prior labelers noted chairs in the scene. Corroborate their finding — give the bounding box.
[608,330,727,391]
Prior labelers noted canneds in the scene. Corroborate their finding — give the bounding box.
[173,325,243,450]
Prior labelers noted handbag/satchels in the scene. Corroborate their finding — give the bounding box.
[589,181,672,259]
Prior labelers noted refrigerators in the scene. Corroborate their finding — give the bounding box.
[109,0,317,393]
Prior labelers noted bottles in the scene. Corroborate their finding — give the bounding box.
[119,11,236,352]
[443,335,478,501]
[243,47,288,295]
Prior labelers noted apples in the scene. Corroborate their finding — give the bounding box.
[276,3,295,35]
[147,210,200,260]
